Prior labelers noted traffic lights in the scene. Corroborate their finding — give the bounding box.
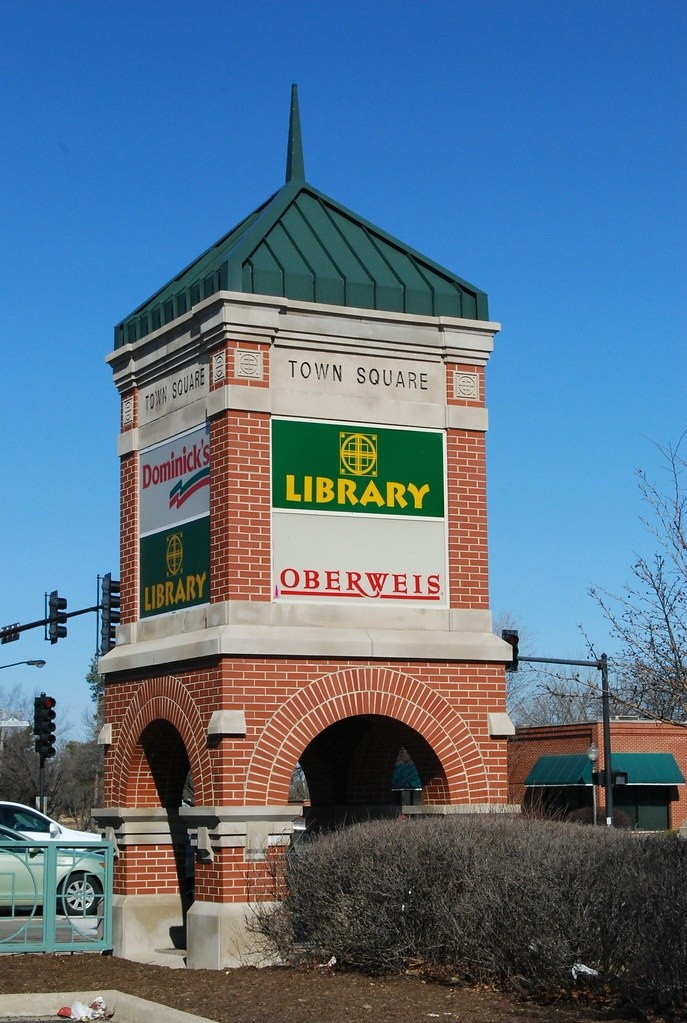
[48,589,69,644]
[36,733,56,759]
[501,629,519,672]
[614,770,628,784]
[98,573,120,655]
[590,769,603,785]
[34,695,56,734]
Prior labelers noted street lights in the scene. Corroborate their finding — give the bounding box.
[587,742,598,826]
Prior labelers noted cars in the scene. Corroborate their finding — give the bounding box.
[0,801,103,854]
[0,823,104,916]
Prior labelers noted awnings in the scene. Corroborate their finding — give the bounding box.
[523,753,686,787]
